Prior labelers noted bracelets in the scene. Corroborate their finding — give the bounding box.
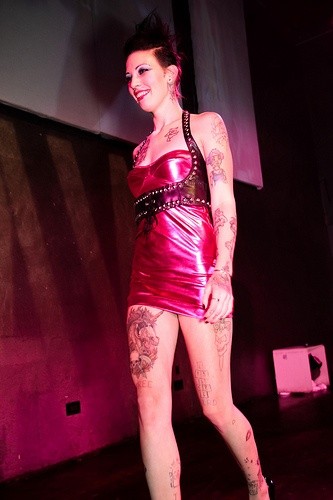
[214,270,232,278]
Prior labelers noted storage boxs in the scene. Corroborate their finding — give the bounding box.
[273,344,330,400]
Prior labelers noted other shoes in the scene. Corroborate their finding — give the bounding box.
[265,477,275,500]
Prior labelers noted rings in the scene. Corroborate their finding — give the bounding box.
[213,298,219,302]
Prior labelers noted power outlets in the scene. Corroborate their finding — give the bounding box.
[65,401,81,416]
[172,380,184,391]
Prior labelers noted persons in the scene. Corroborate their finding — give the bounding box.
[124,9,270,500]
